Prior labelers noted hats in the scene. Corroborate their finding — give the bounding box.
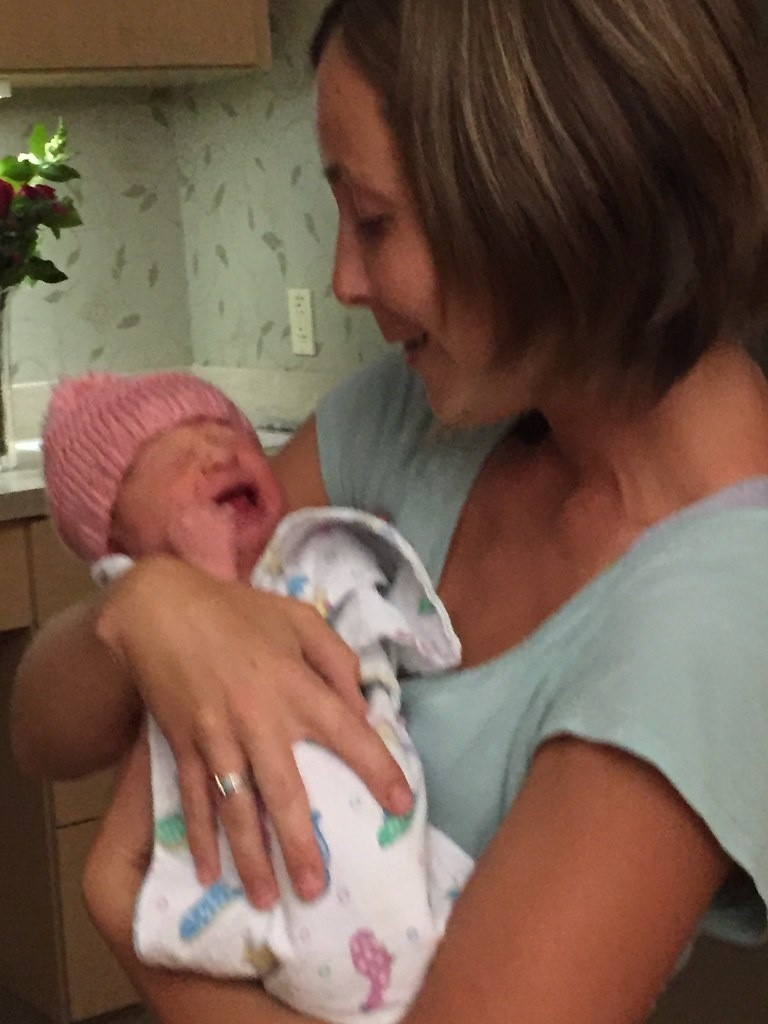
[39,370,269,564]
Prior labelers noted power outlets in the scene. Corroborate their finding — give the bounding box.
[287,289,317,358]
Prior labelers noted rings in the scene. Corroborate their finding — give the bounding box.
[209,771,252,802]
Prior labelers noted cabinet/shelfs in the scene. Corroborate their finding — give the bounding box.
[0,441,310,1023]
[1,1,274,93]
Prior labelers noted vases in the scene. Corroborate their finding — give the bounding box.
[0,284,22,470]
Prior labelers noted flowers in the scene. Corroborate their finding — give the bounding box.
[0,111,84,308]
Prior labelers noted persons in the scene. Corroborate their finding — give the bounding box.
[43,372,474,1024]
[10,0,768,1024]
